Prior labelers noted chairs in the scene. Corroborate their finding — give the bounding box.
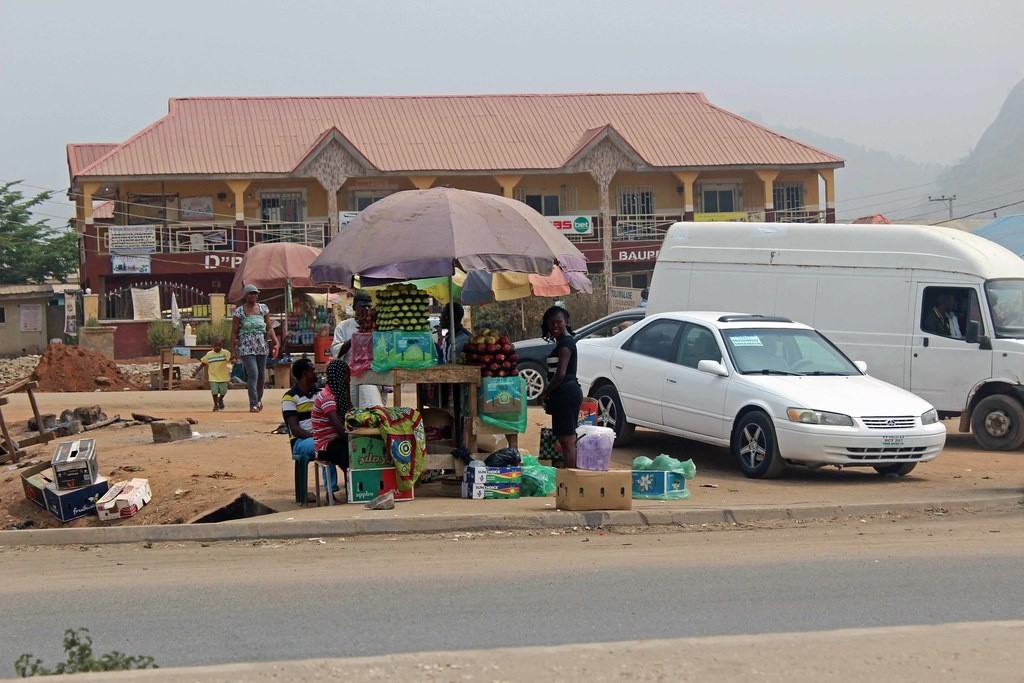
[685,332,715,368]
[283,422,314,508]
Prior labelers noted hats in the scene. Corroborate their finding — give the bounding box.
[353,294,372,309]
[240,285,260,298]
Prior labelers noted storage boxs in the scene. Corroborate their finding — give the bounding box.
[345,433,415,503]
[373,331,432,366]
[19,438,152,523]
[478,376,523,413]
[461,466,521,499]
[557,397,686,511]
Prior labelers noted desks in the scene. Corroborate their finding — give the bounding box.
[158,345,213,390]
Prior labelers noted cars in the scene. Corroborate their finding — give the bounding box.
[508,306,647,407]
[573,308,948,481]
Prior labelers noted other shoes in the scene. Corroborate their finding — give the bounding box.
[219,399,225,411]
[249,402,263,412]
[213,404,219,412]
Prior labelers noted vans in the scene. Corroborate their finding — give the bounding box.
[642,218,1024,451]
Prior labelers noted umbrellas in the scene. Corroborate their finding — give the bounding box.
[354,262,594,306]
[228,242,356,309]
[308,186,589,364]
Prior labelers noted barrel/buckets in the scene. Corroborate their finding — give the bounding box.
[573,425,617,470]
[184,335,196,346]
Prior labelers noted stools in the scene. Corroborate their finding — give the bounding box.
[314,460,342,506]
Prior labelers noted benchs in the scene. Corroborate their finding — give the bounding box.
[633,331,671,359]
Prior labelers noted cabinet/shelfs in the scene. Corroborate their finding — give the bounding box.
[349,365,519,478]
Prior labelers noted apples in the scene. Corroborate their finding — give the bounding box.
[356,284,430,331]
[462,328,521,377]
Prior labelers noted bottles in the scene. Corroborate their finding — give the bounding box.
[287,298,337,344]
[185,323,191,334]
[192,326,197,335]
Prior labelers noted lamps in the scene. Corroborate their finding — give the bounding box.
[676,186,683,195]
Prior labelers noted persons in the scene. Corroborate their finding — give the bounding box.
[537,306,583,468]
[924,293,950,336]
[439,303,474,442]
[970,290,1000,335]
[229,285,280,412]
[637,289,649,307]
[619,321,633,331]
[331,294,388,410]
[191,337,236,411]
[311,359,354,503]
[281,358,343,505]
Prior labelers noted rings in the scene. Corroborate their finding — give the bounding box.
[542,397,544,399]
